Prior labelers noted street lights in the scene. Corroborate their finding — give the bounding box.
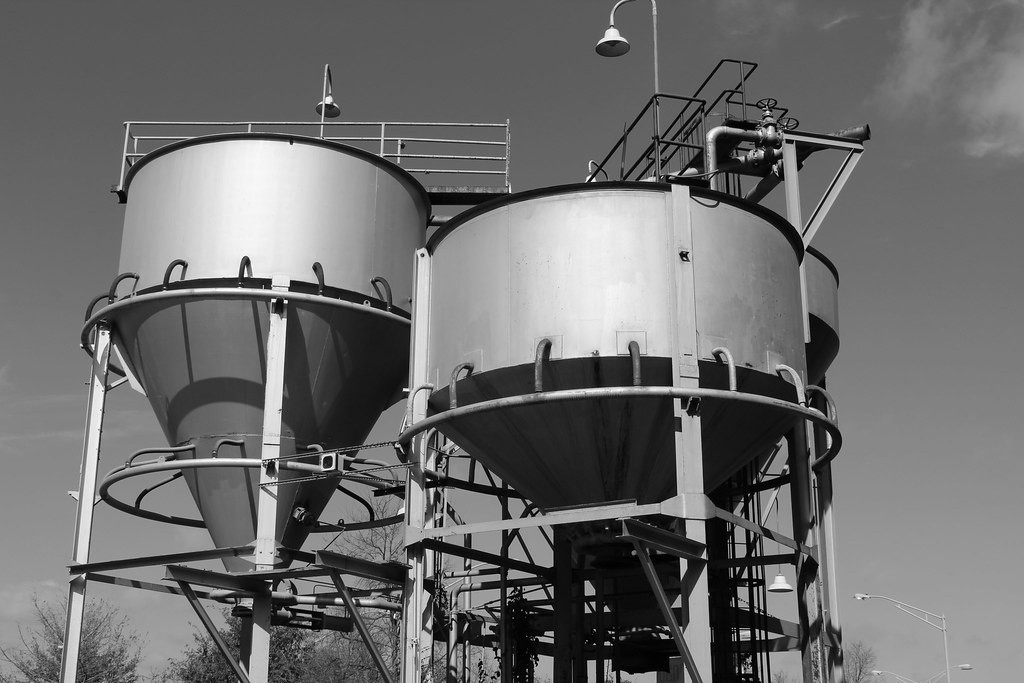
[854,593,951,683]
